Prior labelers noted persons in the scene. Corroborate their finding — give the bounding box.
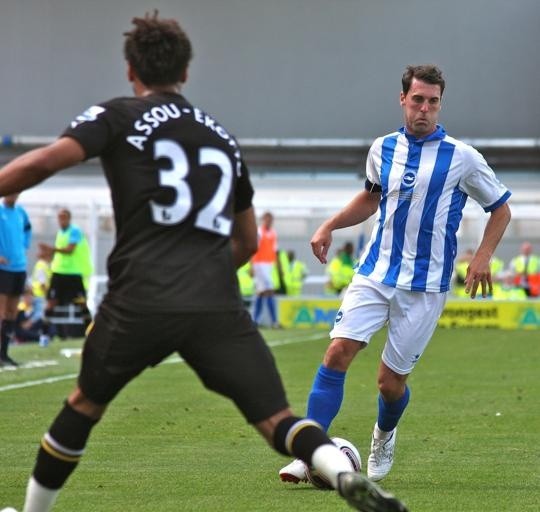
[453,245,540,301]
[1,7,412,512]
[236,212,360,330]
[0,189,94,368]
[272,61,518,488]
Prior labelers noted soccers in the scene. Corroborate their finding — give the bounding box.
[305,437,361,489]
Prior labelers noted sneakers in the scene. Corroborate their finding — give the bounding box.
[278,457,311,484]
[338,471,410,512]
[366,421,398,483]
